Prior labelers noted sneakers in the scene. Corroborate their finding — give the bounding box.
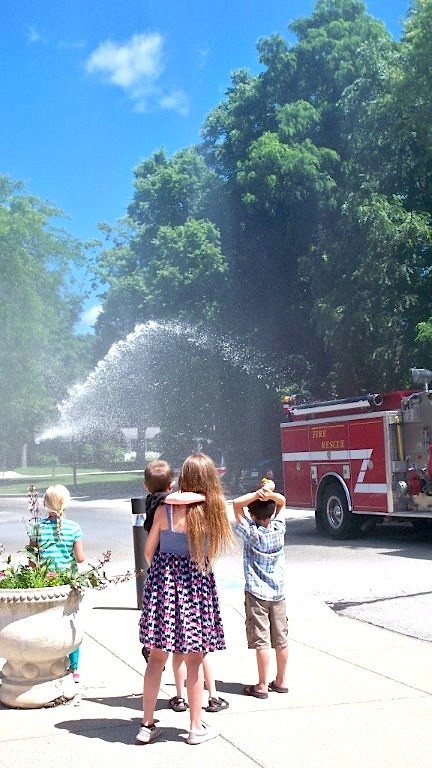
[135,726,162,743]
[186,724,219,745]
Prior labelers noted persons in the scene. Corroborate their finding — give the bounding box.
[28,484,84,683]
[136,453,221,744]
[142,459,205,671]
[170,652,230,711]
[234,481,288,699]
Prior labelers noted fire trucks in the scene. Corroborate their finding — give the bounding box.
[278,368,432,541]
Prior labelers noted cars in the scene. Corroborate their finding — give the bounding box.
[156,447,283,495]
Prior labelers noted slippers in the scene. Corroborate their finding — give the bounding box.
[243,684,268,699]
[269,680,289,694]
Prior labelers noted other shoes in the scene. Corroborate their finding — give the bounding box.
[142,646,167,673]
[68,670,80,683]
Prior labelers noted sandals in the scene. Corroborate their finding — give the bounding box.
[207,696,229,712]
[168,696,186,711]
